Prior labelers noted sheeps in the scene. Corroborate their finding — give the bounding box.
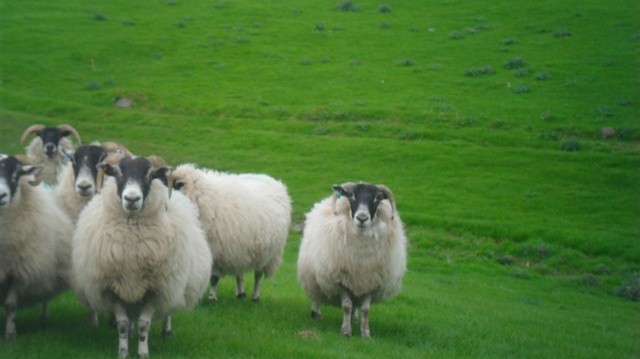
[0,154,75,339]
[57,141,128,329]
[72,154,213,359]
[20,124,82,187]
[159,163,291,304]
[298,182,407,337]
